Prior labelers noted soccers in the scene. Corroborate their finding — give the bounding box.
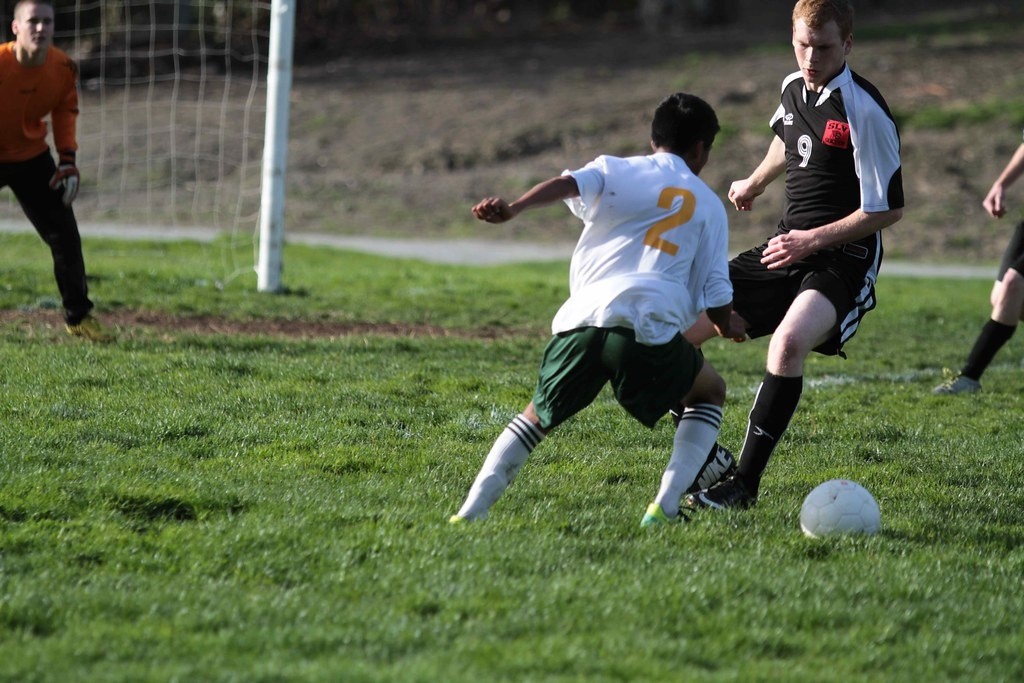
[800,479,881,539]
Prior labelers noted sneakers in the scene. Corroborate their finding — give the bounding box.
[931,374,981,396]
[685,473,755,515]
[684,446,733,496]
[64,313,116,343]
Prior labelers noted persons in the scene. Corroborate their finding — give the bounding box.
[671,0,905,514]
[449,93,745,523]
[0,0,120,343]
[932,142,1023,394]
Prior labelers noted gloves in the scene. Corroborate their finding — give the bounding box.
[49,150,80,206]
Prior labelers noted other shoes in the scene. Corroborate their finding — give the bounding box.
[639,501,696,528]
